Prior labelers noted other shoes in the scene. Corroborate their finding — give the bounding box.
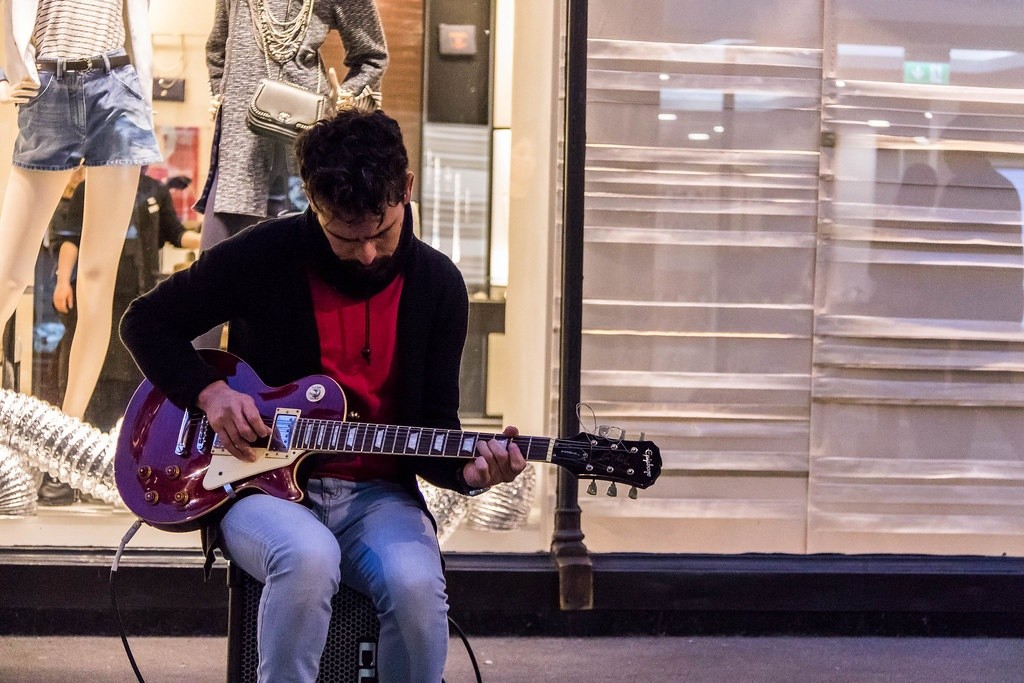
[38,473,74,506]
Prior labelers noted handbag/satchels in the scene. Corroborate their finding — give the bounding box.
[153,78,185,102]
[246,78,325,145]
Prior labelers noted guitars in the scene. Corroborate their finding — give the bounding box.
[114,347,663,533]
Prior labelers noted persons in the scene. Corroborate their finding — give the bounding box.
[0,1,388,508]
[118,110,528,683]
[51,164,202,433]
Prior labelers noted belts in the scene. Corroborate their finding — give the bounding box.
[33,55,131,71]
